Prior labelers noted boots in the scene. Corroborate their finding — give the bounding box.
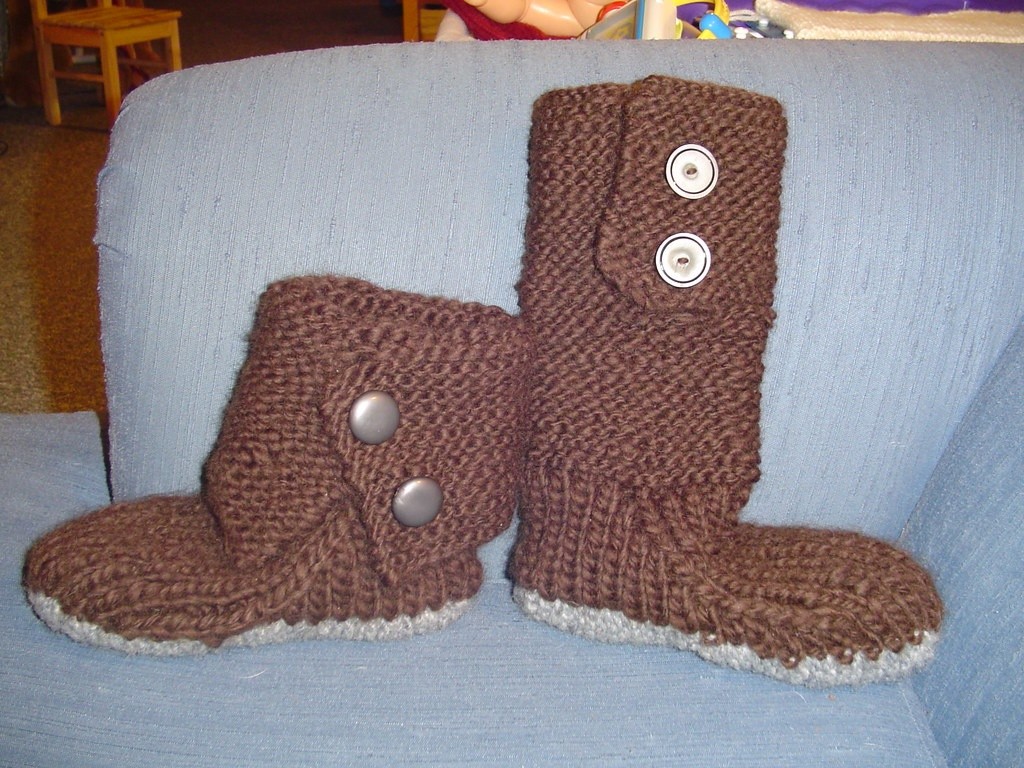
[22,275,532,660]
[505,74,943,687]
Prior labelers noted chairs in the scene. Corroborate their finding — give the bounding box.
[30,0,183,131]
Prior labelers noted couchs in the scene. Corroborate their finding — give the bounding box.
[0,39,1024,768]
